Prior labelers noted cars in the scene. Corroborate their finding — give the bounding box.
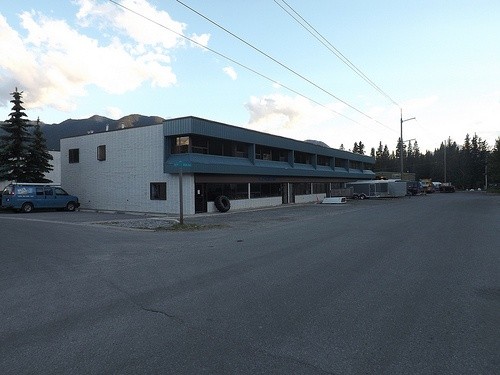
[420,180,436,193]
[438,182,456,193]
[408,181,422,194]
[431,182,441,190]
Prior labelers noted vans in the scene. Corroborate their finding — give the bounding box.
[1,182,81,213]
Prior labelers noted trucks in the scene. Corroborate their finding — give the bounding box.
[388,178,407,197]
[343,181,388,199]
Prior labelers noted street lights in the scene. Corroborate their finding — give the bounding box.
[401,116,415,180]
